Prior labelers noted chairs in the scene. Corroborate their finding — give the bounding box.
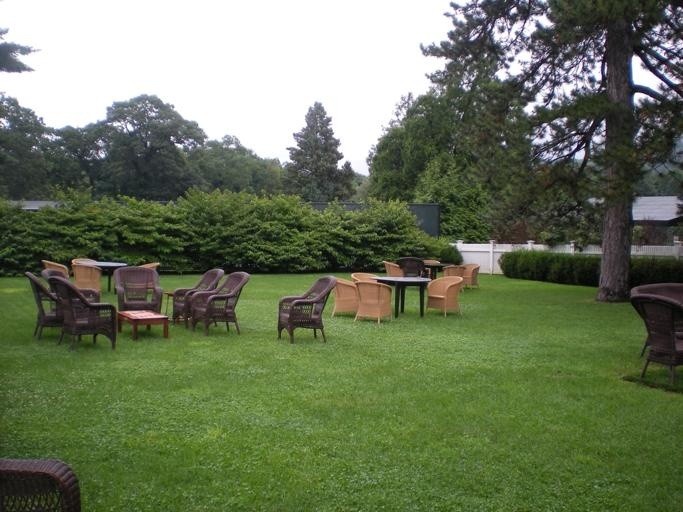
[276,275,333,345]
[629,295,683,385]
[25,258,251,351]
[0,459,79,512]
[630,283,683,326]
[334,256,481,325]
